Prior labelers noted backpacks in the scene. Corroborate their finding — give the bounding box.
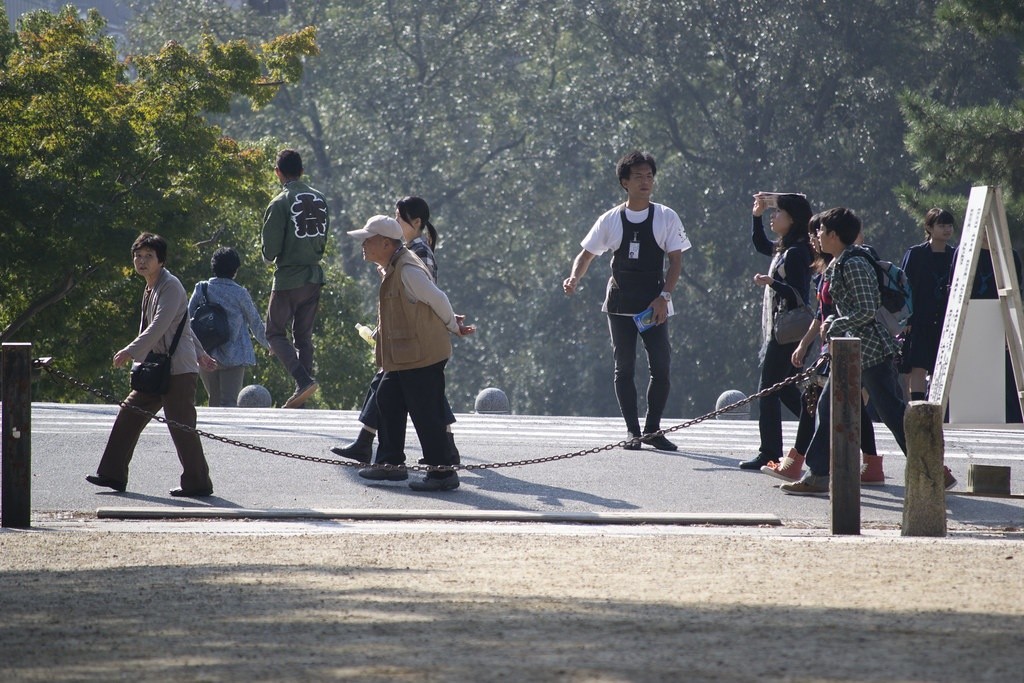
[190,282,229,354]
[838,249,913,335]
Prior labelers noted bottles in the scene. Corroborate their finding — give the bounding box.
[355,323,377,347]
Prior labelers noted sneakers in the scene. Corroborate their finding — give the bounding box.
[943,466,958,490]
[780,469,830,497]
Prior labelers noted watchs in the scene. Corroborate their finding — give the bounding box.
[660,291,672,301]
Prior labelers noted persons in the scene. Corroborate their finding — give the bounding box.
[330,195,462,466]
[759,213,884,486]
[85,231,219,498]
[894,208,1023,423]
[260,150,330,409]
[358,214,476,493]
[563,151,682,452]
[739,195,818,467]
[187,247,274,407]
[781,208,958,492]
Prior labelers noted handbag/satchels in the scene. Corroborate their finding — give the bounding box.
[131,353,172,395]
[774,285,816,345]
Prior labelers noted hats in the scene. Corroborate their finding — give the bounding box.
[347,214,403,240]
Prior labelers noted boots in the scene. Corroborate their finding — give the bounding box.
[760,448,805,483]
[418,432,460,466]
[330,427,376,464]
[860,453,885,486]
[911,392,925,401]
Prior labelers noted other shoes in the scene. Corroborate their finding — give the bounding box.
[624,428,641,450]
[358,463,408,481]
[408,465,460,492]
[168,487,213,498]
[642,426,678,451]
[739,453,780,470]
[85,474,126,492]
[282,376,318,408]
